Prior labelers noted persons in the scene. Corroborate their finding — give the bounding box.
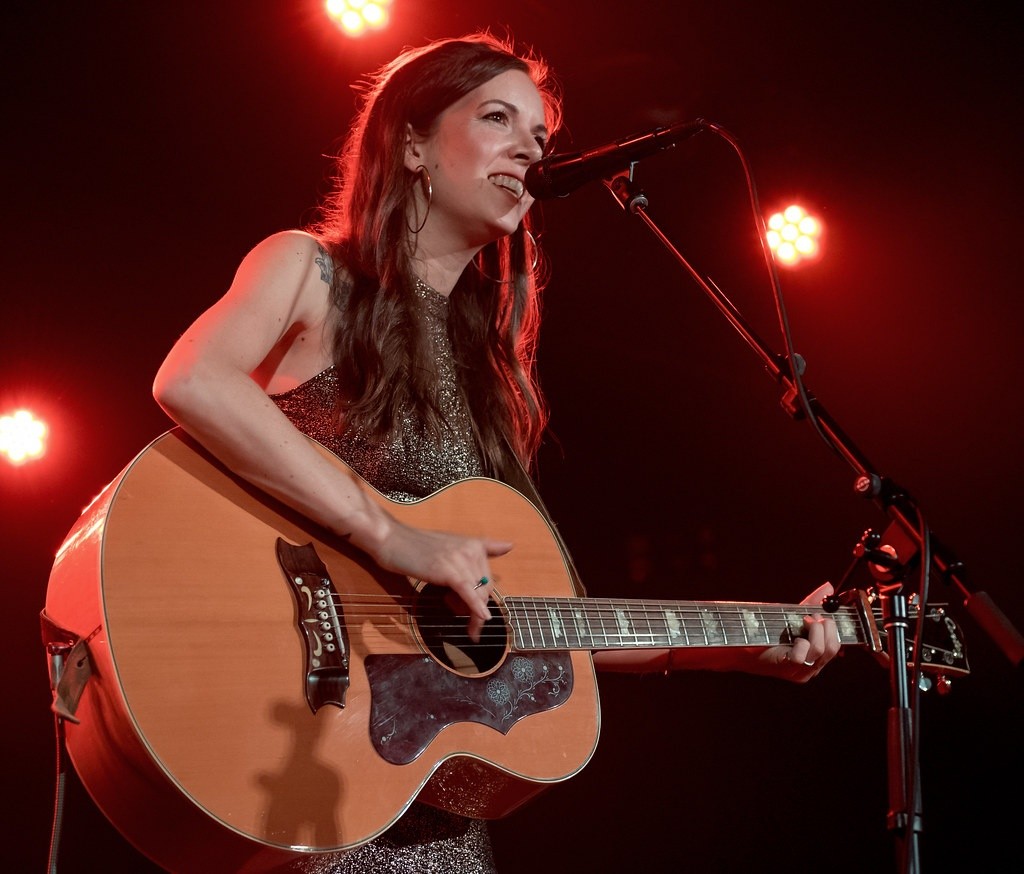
[152,37,842,874]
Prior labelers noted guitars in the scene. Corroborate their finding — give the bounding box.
[41,419,975,874]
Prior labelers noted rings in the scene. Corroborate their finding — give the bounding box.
[475,577,488,589]
[803,661,814,666]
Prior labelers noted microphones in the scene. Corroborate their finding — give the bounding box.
[524,118,706,201]
